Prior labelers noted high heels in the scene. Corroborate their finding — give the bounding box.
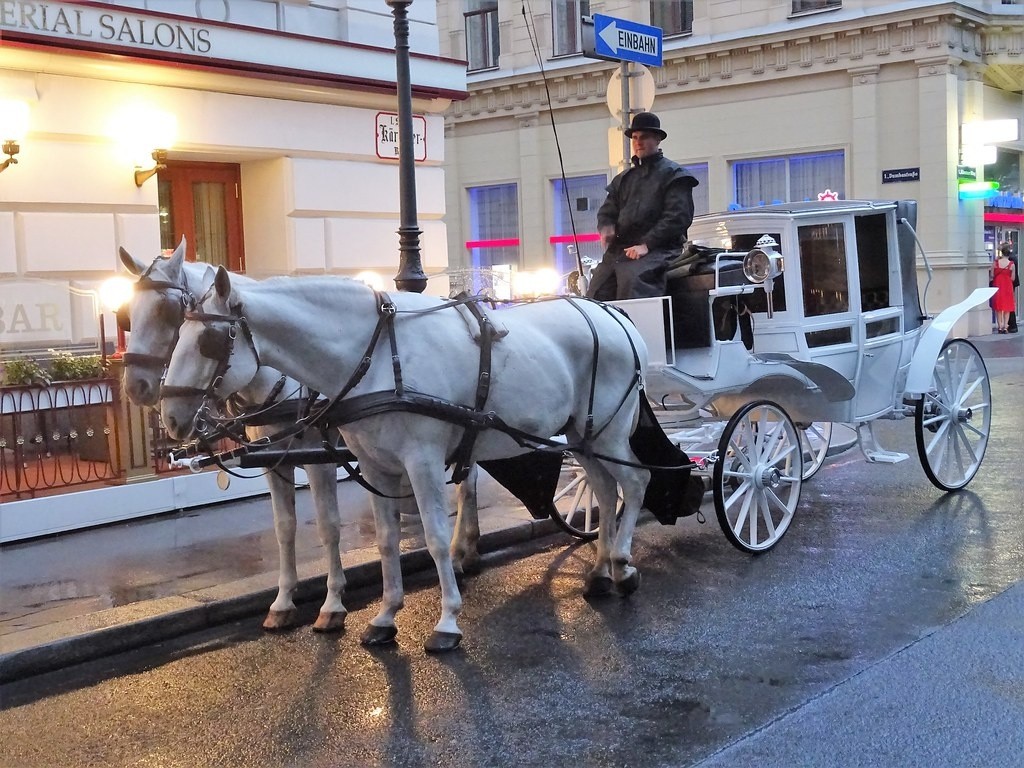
[998,327,1008,334]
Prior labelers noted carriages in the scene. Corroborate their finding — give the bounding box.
[115,198,998,652]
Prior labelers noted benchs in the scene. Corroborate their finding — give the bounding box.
[663,251,755,350]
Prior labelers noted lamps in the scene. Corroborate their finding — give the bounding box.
[567,256,595,297]
[744,233,787,320]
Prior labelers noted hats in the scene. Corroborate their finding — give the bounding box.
[625,112,667,141]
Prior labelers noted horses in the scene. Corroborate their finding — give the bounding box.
[117,234,653,655]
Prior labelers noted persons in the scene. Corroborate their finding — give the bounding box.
[585,111,699,300]
[991,242,1020,334]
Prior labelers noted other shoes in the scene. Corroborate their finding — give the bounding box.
[1009,328,1018,333]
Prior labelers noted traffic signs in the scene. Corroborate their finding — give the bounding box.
[593,12,664,69]
